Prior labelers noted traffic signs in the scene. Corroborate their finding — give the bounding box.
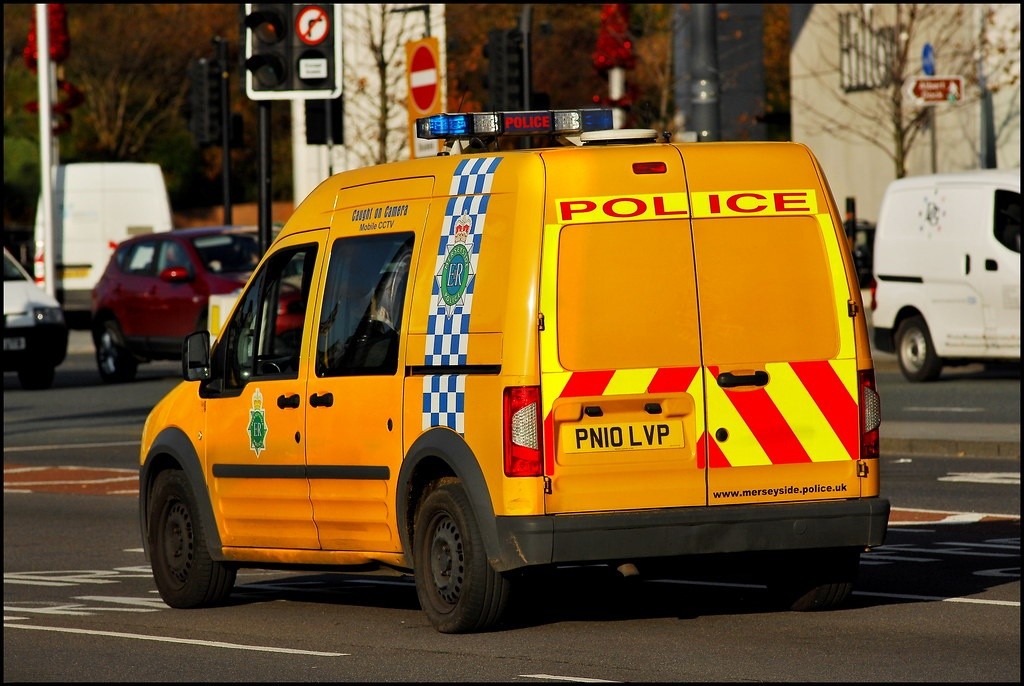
[903,75,966,107]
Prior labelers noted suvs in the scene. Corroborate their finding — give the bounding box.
[90,224,305,384]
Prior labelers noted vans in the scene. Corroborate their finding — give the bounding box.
[869,167,1021,385]
[136,108,888,635]
[31,163,174,324]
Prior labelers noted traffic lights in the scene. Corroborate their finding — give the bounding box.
[242,2,346,100]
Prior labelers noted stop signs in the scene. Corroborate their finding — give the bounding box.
[401,37,444,119]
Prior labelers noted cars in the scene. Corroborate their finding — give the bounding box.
[3,248,70,392]
